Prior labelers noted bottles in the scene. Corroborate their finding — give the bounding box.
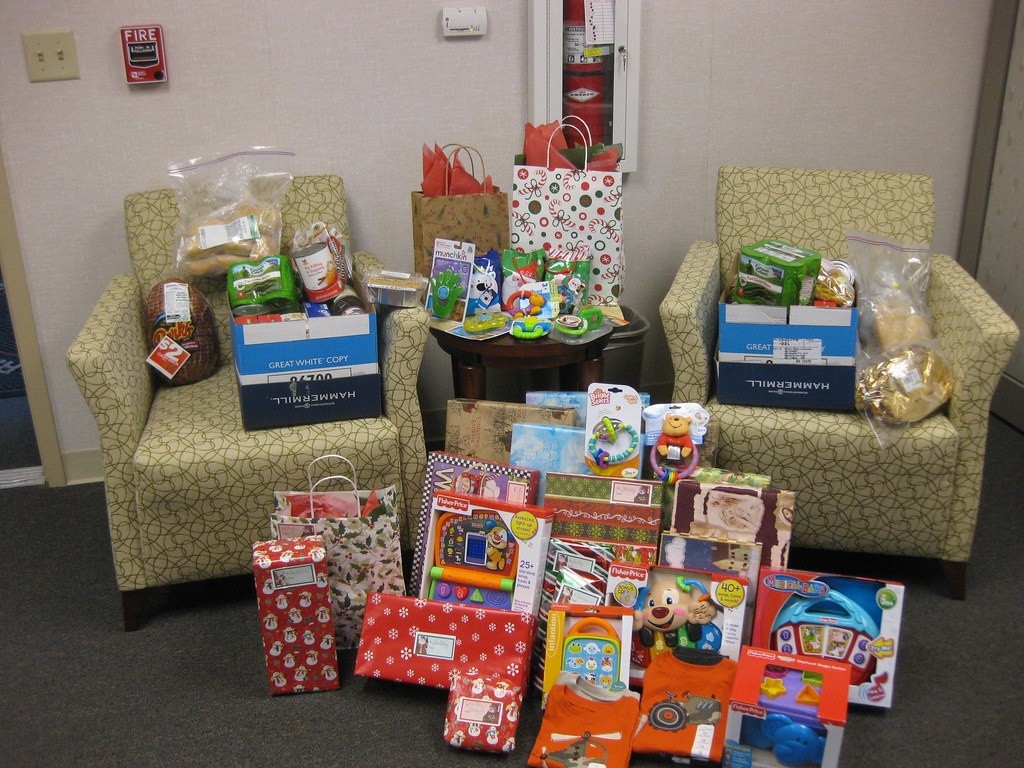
[328,282,366,315]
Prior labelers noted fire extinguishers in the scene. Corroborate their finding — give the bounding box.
[563,0,605,148]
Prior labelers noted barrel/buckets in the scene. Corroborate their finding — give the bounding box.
[831,259,855,286]
[227,242,345,318]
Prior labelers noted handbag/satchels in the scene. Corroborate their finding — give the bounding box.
[512,115,625,307]
[411,144,510,305]
[270,454,406,651]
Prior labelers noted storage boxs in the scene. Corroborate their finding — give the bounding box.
[227,250,906,768]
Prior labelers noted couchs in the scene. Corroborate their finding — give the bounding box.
[659,166,1020,600]
[67,175,432,634]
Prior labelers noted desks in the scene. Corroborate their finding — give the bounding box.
[429,326,618,402]
